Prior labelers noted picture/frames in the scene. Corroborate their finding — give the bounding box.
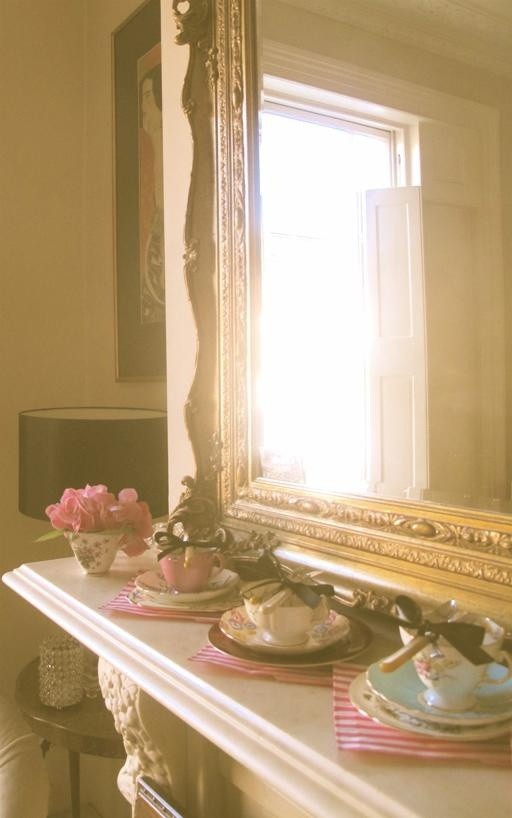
[109,1,167,383]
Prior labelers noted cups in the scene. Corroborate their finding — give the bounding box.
[37,634,86,710]
[398,610,512,713]
[239,577,329,646]
[155,546,223,593]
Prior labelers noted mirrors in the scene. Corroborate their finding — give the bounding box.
[169,0,512,642]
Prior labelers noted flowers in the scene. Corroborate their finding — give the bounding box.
[42,483,154,558]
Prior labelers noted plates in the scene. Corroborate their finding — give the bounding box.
[348,653,512,742]
[125,566,248,613]
[207,603,375,668]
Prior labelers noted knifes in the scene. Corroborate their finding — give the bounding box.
[381,611,479,673]
[259,570,324,615]
